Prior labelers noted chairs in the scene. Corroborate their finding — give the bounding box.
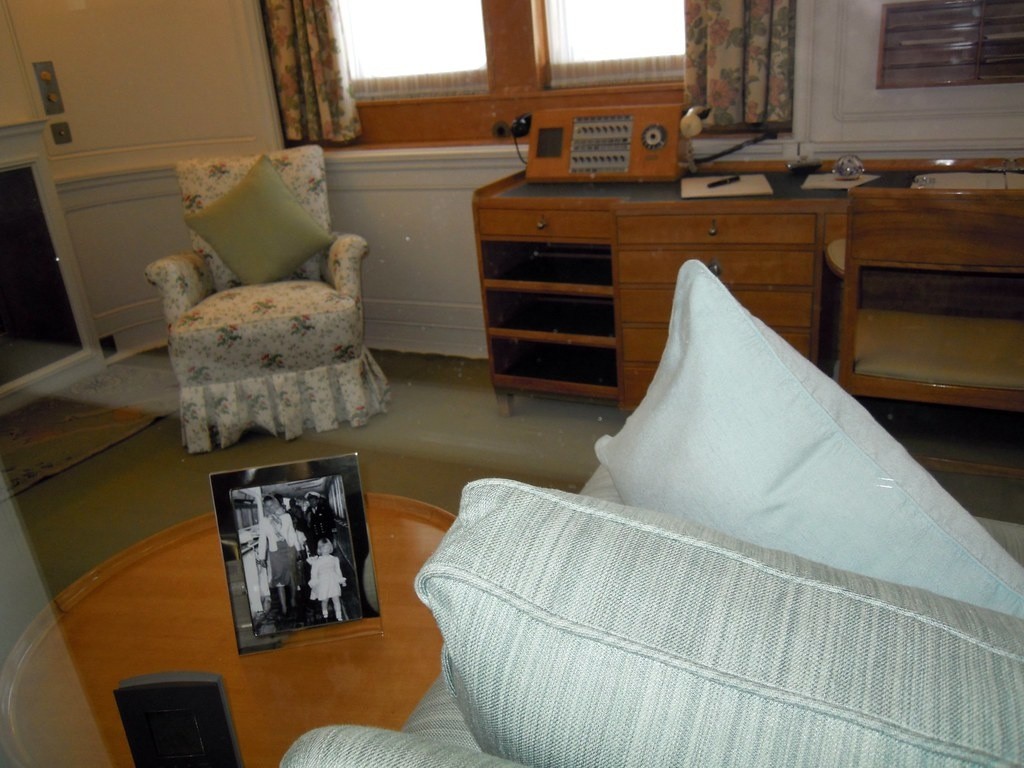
[824,183,1024,495]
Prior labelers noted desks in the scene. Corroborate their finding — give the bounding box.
[460,150,1024,428]
[1,493,463,768]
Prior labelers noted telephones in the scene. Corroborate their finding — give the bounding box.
[511,103,711,182]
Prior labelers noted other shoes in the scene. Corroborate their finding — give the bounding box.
[322,611,329,622]
[337,617,344,621]
[291,603,297,611]
[281,607,289,618]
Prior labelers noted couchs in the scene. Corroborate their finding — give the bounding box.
[276,465,1024,767]
[142,139,394,457]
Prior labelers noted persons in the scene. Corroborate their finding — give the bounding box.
[257,490,348,623]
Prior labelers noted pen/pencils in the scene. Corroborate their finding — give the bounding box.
[707,176,739,188]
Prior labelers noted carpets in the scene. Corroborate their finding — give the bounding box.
[0,354,182,498]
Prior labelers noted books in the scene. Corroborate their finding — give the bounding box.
[681,173,775,199]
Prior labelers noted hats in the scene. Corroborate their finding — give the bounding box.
[304,491,320,500]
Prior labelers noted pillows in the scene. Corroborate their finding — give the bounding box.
[588,256,1023,635]
[183,150,329,289]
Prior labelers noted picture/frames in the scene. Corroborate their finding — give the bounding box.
[204,452,388,658]
